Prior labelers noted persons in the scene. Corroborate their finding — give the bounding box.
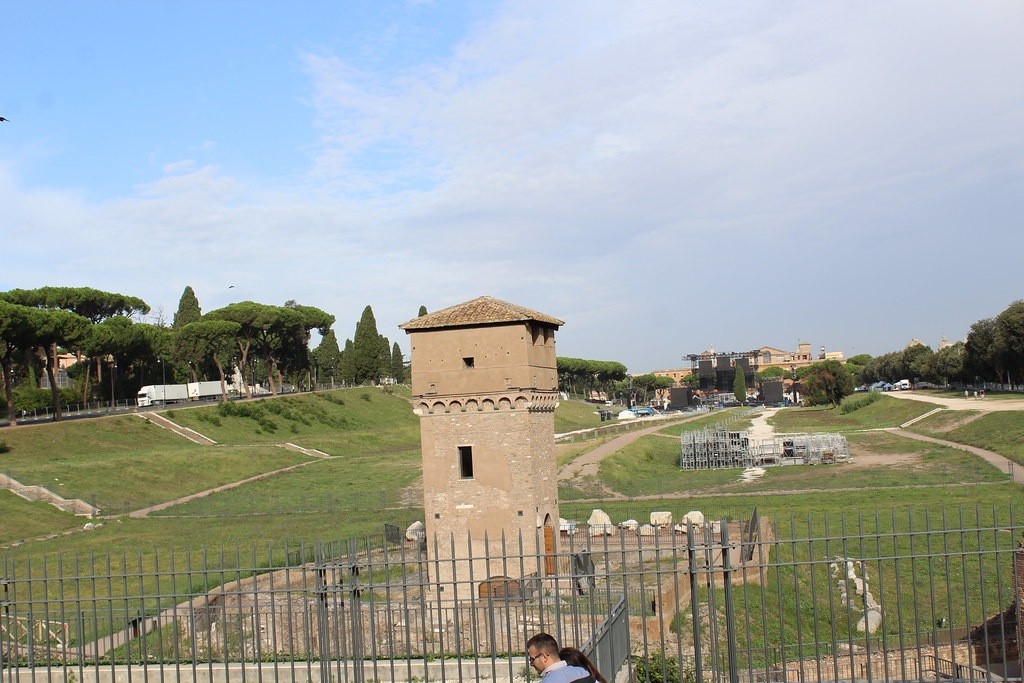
[965,390,985,401]
[521,633,610,683]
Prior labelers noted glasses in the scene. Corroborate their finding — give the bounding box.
[530,654,549,663]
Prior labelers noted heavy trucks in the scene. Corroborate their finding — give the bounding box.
[137,385,188,408]
